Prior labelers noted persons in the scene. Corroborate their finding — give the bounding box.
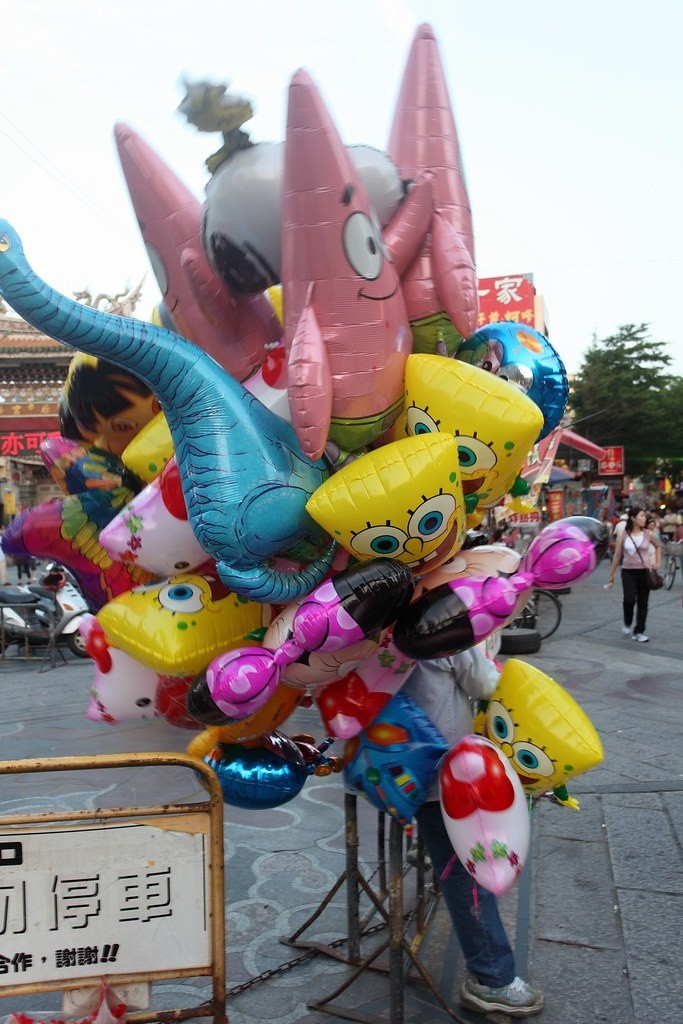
[16,555,38,585]
[475,522,519,547]
[414,804,544,1016]
[608,507,661,642]
[604,500,683,569]
[0,522,11,585]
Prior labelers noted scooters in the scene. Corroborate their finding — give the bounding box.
[0,558,102,658]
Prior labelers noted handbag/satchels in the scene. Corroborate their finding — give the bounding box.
[649,570,663,590]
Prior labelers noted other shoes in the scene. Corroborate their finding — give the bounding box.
[33,577,37,581]
[17,582,22,585]
[27,582,31,584]
[3,583,11,586]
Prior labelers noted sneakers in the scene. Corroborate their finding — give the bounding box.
[622,626,631,635]
[460,974,544,1017]
[631,633,649,642]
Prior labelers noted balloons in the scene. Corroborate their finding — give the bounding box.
[0,21,604,895]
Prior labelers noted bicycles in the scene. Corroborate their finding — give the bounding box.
[507,587,563,641]
[660,535,683,590]
